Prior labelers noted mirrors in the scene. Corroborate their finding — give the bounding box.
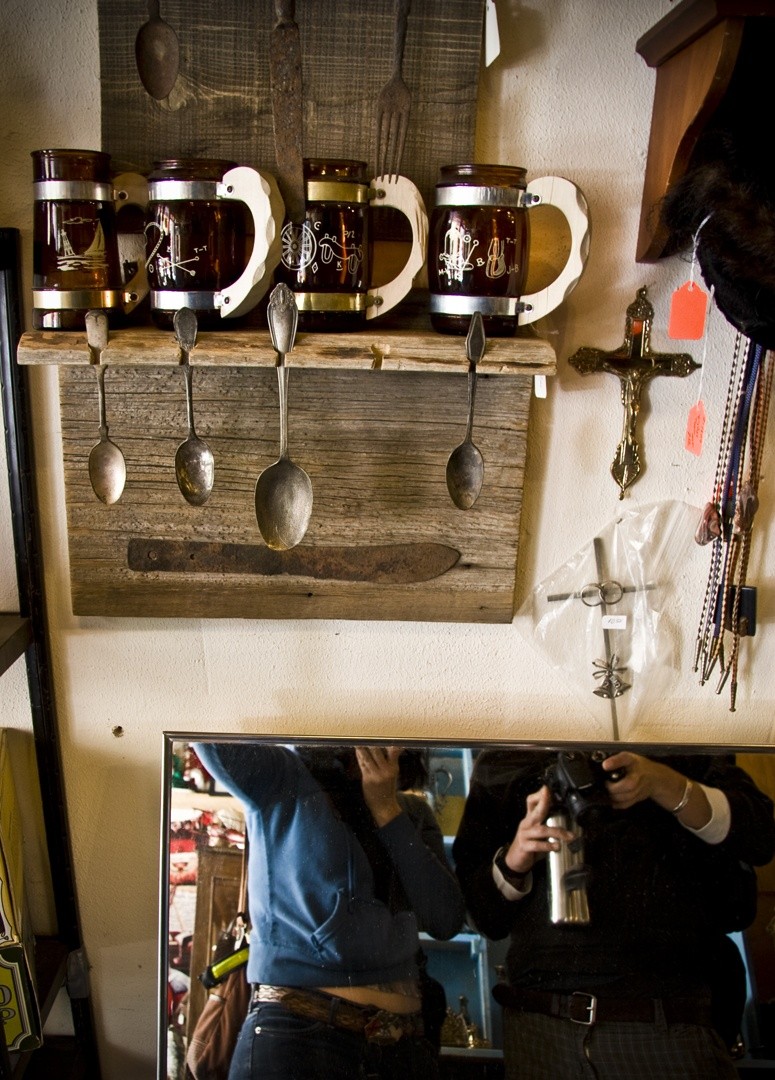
[156,728,775,1080]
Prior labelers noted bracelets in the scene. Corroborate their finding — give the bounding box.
[670,778,694,814]
[378,808,401,829]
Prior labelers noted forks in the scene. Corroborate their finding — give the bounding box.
[375,0,411,184]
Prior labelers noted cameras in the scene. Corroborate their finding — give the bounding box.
[538,754,626,832]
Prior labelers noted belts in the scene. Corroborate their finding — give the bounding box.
[256,985,427,1044]
[521,991,716,1025]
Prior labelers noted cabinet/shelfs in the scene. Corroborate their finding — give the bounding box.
[0,228,109,1080]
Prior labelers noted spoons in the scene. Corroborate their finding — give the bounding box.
[254,282,313,552]
[446,311,486,511]
[86,309,125,505]
[134,0,178,100]
[172,307,214,505]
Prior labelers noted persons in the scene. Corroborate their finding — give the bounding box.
[189,740,465,1080]
[452,748,775,1080]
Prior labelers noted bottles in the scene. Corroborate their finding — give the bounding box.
[547,798,589,924]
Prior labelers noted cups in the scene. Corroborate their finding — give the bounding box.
[30,149,150,331]
[427,162,592,334]
[146,160,276,331]
[269,158,429,334]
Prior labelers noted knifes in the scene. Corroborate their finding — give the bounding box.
[268,0,307,223]
[127,537,460,584]
